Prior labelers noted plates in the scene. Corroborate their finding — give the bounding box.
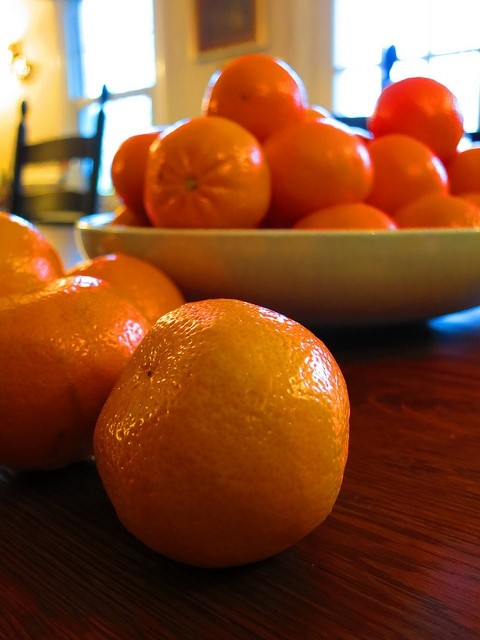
[71,210,480,331]
